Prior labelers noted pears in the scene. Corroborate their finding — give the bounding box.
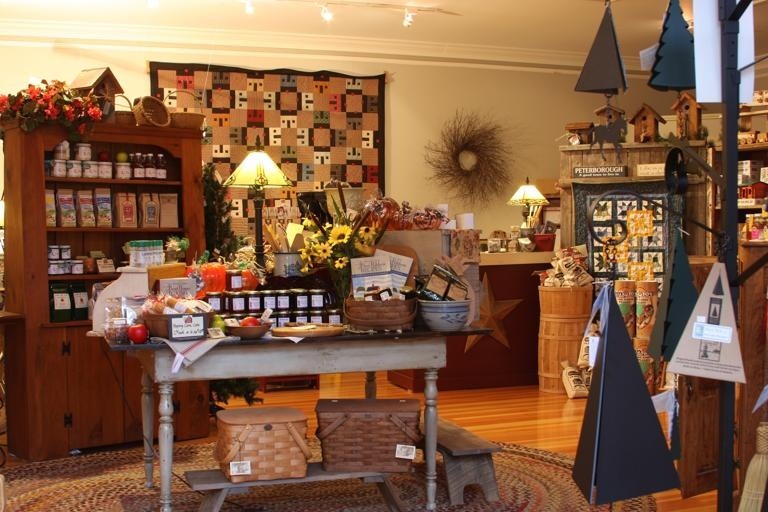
[212,315,225,335]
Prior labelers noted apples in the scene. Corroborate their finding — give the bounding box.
[128,324,147,344]
[242,317,259,327]
[117,153,128,162]
[99,152,110,162]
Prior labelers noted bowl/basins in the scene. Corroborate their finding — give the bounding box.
[141,311,216,338]
[416,297,472,333]
[223,318,273,339]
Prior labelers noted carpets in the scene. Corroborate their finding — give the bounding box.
[3,443,656,511]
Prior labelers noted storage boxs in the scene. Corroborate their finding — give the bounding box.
[344,291,415,333]
[317,397,426,474]
[212,405,312,483]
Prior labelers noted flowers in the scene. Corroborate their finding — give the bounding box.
[0,79,105,142]
[299,180,390,295]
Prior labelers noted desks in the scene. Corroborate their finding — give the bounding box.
[140,336,447,512]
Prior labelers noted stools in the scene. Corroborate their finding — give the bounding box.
[186,462,411,511]
[418,416,501,508]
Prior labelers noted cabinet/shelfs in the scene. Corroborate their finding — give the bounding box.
[712,108,768,265]
[5,112,205,463]
[557,138,708,307]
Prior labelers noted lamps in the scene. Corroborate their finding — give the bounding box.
[508,178,547,230]
[223,135,297,268]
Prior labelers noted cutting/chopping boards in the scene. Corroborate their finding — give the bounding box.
[271,326,348,337]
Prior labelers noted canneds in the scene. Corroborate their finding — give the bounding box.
[326,309,343,323]
[205,292,223,312]
[54,143,70,160]
[268,312,277,330]
[277,312,292,327]
[60,245,71,259]
[226,270,242,291]
[48,245,59,260]
[83,161,99,178]
[310,310,326,323]
[223,292,231,312]
[58,261,65,274]
[98,162,112,178]
[72,260,83,274]
[292,311,309,323]
[310,289,326,309]
[292,289,310,309]
[230,292,247,313]
[48,261,59,275]
[246,291,263,312]
[262,290,277,311]
[66,160,82,177]
[275,290,291,310]
[64,260,72,274]
[74,143,91,160]
[53,160,66,177]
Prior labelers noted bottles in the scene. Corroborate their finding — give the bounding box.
[133,153,145,179]
[104,318,130,343]
[156,154,167,180]
[145,154,156,179]
[115,162,130,178]
